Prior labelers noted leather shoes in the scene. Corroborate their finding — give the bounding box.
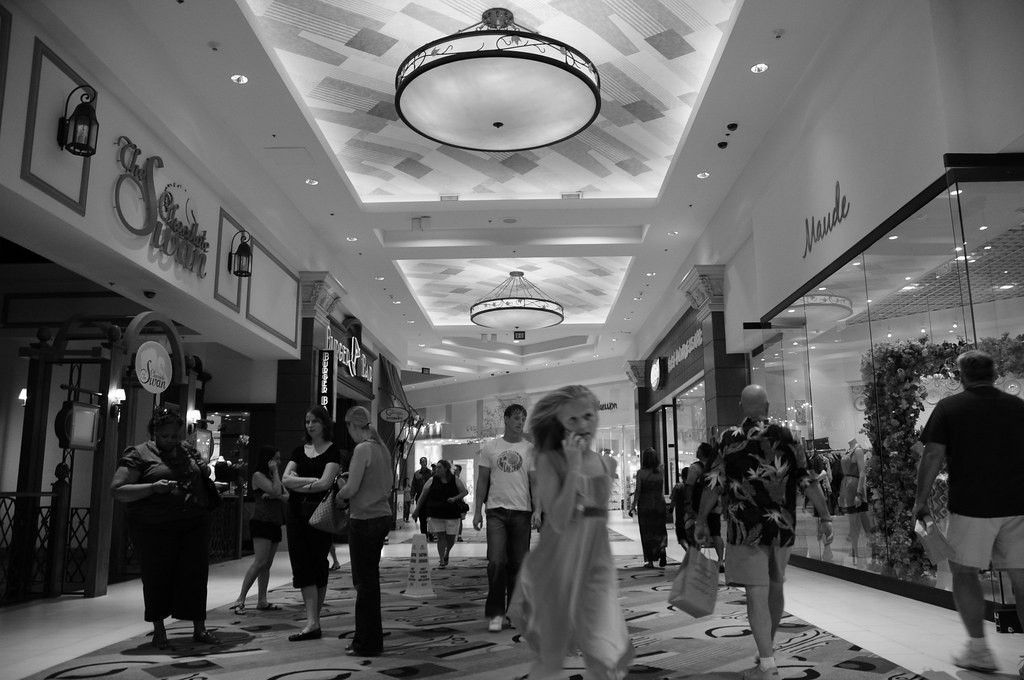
[289,628,321,641]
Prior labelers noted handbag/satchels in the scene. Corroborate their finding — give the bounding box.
[450,499,469,518]
[309,472,353,538]
[178,472,223,524]
[668,539,719,619]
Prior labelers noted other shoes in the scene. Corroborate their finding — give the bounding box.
[660,550,667,567]
[508,617,517,628]
[444,557,448,565]
[488,615,503,631]
[344,644,380,656]
[719,565,725,573]
[329,564,340,570]
[643,564,653,568]
[439,561,445,568]
[457,537,463,542]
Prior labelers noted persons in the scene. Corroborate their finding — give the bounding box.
[280,405,342,641]
[229,442,727,615]
[802,437,880,561]
[518,384,634,680]
[908,351,1024,680]
[694,384,835,680]
[334,405,393,657]
[473,404,541,633]
[111,405,219,651]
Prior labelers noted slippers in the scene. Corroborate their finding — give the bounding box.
[235,604,246,615]
[256,603,283,610]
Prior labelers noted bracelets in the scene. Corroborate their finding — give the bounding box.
[820,518,832,522]
[150,483,156,493]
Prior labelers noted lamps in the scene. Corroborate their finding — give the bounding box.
[58,84,99,156]
[228,230,253,277]
[394,7,601,151]
[188,410,201,434]
[470,271,565,331]
[110,388,126,419]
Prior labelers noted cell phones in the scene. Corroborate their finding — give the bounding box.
[168,481,178,486]
[551,417,570,437]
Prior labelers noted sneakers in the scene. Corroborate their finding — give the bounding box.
[742,665,780,680]
[951,640,997,674]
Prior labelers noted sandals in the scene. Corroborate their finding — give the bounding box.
[194,631,220,644]
[152,631,167,649]
